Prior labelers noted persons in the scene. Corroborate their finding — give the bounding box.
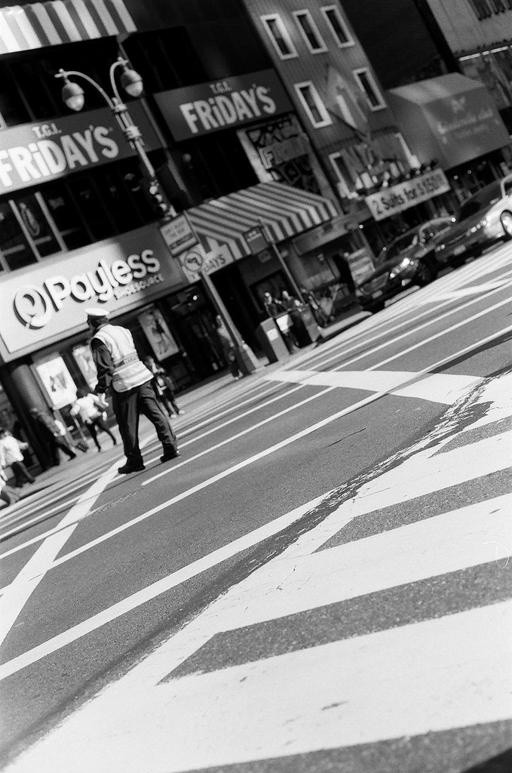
[0,428,36,488]
[300,286,329,328]
[143,354,186,418]
[282,290,297,310]
[263,291,301,353]
[215,314,242,380]
[0,477,20,507]
[69,388,117,452]
[29,406,76,467]
[83,306,181,473]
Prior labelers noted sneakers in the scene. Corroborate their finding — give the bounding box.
[159,451,180,462]
[118,463,146,474]
[169,409,186,418]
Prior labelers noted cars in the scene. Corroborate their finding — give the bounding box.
[434,171,512,272]
[353,213,460,318]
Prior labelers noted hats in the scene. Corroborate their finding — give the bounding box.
[84,307,108,321]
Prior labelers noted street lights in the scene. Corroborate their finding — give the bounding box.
[49,55,267,378]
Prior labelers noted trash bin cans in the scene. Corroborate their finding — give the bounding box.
[254,303,324,363]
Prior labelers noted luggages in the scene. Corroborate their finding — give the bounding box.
[62,436,90,452]
